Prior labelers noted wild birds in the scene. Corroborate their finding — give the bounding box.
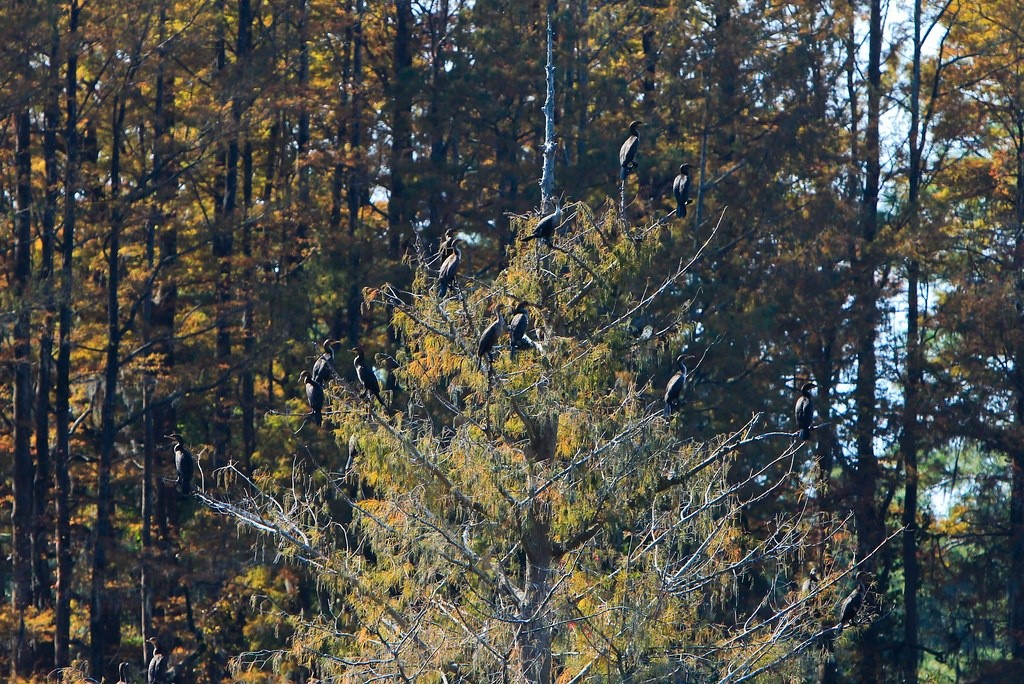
[115,637,167,684]
[165,433,193,497]
[781,558,875,634]
[296,120,823,441]
